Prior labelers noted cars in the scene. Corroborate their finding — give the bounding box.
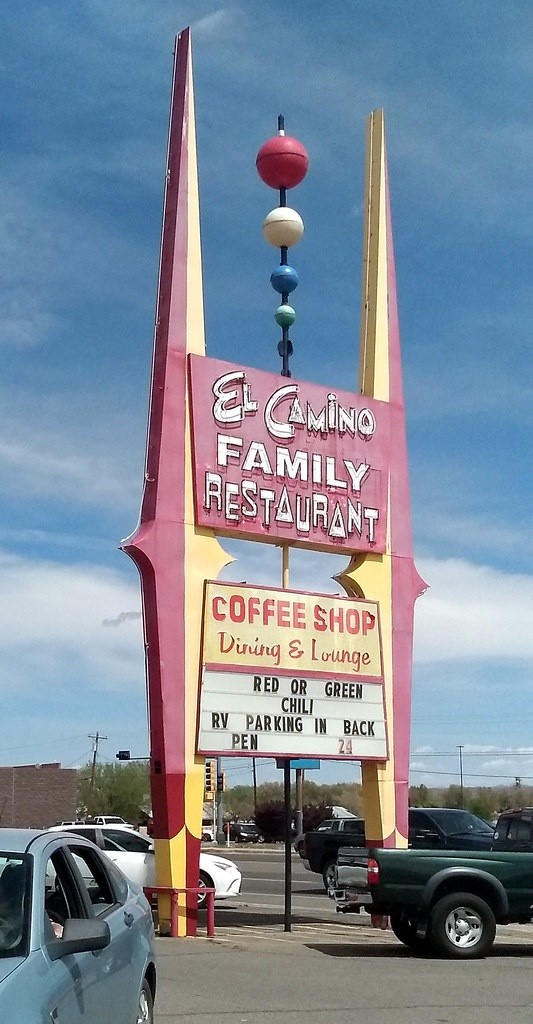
[0,828,157,1024]
[42,815,243,908]
[292,818,364,859]
[223,823,259,844]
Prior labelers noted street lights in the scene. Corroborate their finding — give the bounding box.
[457,746,464,809]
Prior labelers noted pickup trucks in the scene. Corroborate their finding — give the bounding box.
[326,806,533,959]
[302,807,495,889]
[201,825,218,841]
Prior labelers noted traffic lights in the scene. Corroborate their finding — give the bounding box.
[206,761,215,791]
[218,772,225,792]
[116,750,131,760]
